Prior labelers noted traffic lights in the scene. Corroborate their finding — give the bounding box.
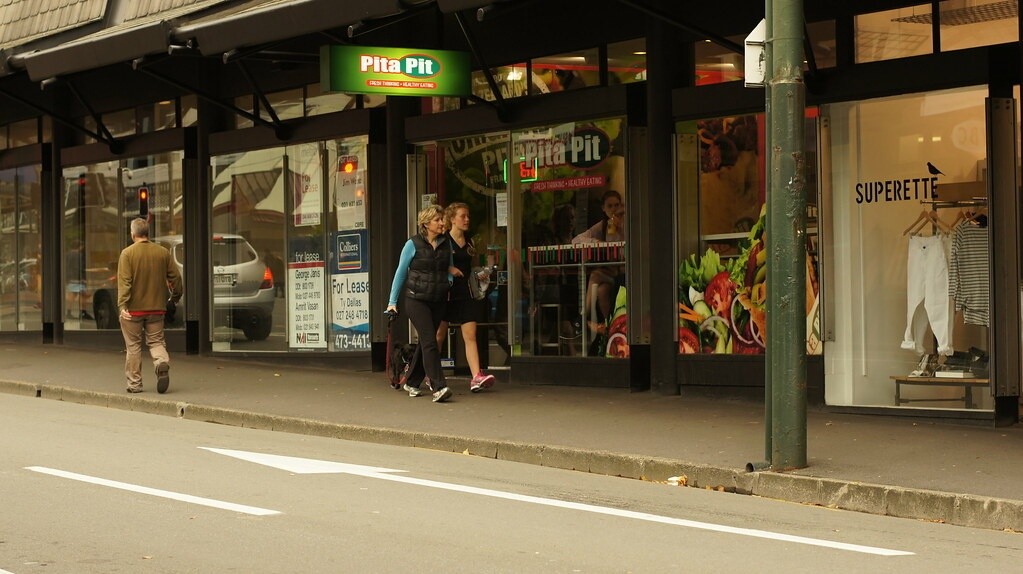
[333,154,360,209]
[138,186,150,218]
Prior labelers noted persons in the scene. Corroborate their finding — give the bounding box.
[117,218,184,393]
[559,191,626,340]
[65,240,93,320]
[425,202,495,393]
[385,205,454,402]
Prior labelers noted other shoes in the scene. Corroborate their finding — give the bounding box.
[126,388,142,393]
[155,362,170,394]
[558,314,587,339]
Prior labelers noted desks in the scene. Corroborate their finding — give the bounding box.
[889,375,991,409]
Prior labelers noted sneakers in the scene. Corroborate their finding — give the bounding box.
[403,383,423,397]
[425,380,434,391]
[470,373,496,394]
[432,387,453,402]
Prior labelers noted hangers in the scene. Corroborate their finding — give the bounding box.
[951,201,989,227]
[903,200,950,236]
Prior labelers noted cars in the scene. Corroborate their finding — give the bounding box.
[33,233,275,341]
